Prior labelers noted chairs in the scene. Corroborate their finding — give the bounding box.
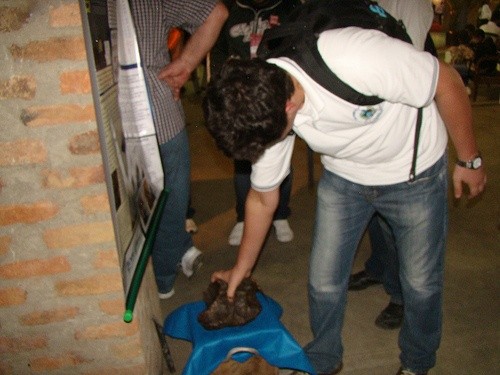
[466,32,500,102]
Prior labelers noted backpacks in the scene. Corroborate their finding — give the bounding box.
[254,0,414,105]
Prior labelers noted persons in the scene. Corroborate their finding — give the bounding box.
[128,0,231,299]
[202,27,488,375]
[156,0,305,246]
[444,18,500,74]
[346,0,437,329]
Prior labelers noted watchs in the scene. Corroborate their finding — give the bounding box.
[456,151,482,171]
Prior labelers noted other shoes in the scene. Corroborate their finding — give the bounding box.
[180,244,203,278]
[373,301,406,330]
[185,217,197,233]
[229,222,244,247]
[346,270,382,291]
[273,218,293,243]
[157,288,175,298]
